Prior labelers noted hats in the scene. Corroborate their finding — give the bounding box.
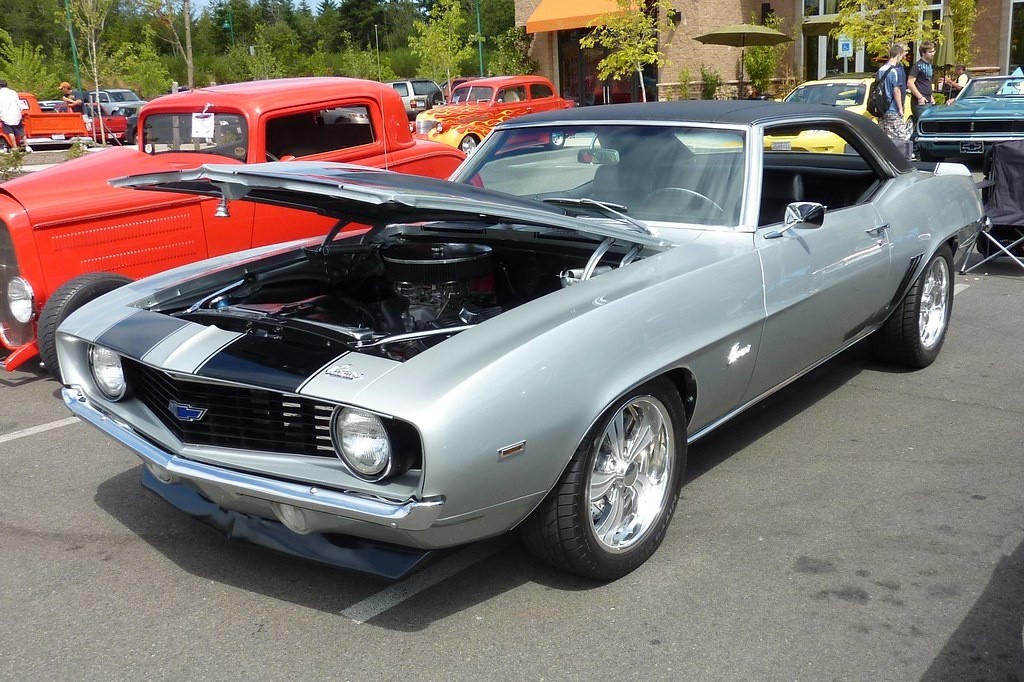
[59,82,69,89]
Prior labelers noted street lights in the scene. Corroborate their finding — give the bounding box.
[222,6,235,47]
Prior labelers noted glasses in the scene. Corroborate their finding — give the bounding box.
[924,50,937,54]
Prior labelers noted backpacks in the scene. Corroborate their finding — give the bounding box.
[867,65,896,119]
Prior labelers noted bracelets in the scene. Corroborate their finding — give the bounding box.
[918,96,923,100]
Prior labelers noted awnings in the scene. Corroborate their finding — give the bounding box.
[526,0,642,35]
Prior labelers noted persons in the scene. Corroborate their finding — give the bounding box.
[907,40,936,161]
[60,81,88,116]
[945,64,971,106]
[746,90,764,100]
[875,44,910,143]
[0,80,34,154]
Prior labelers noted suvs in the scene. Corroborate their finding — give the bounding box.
[86,88,150,118]
[387,80,445,119]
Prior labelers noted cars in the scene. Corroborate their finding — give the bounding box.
[443,76,487,103]
[317,106,370,125]
[721,73,916,164]
[126,109,192,144]
[37,99,66,112]
[910,75,1024,163]
[47,98,997,582]
[415,75,577,160]
[0,76,489,374]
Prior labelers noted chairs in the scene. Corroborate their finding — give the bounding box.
[594,163,651,215]
[750,170,804,224]
[958,139,1024,274]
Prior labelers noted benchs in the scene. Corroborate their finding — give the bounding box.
[657,164,879,218]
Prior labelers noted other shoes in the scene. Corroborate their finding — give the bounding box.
[27,147,33,154]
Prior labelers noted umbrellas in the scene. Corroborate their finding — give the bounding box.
[933,5,955,77]
[691,23,796,99]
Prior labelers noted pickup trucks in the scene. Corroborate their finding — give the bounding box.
[53,102,107,116]
[0,91,127,152]
